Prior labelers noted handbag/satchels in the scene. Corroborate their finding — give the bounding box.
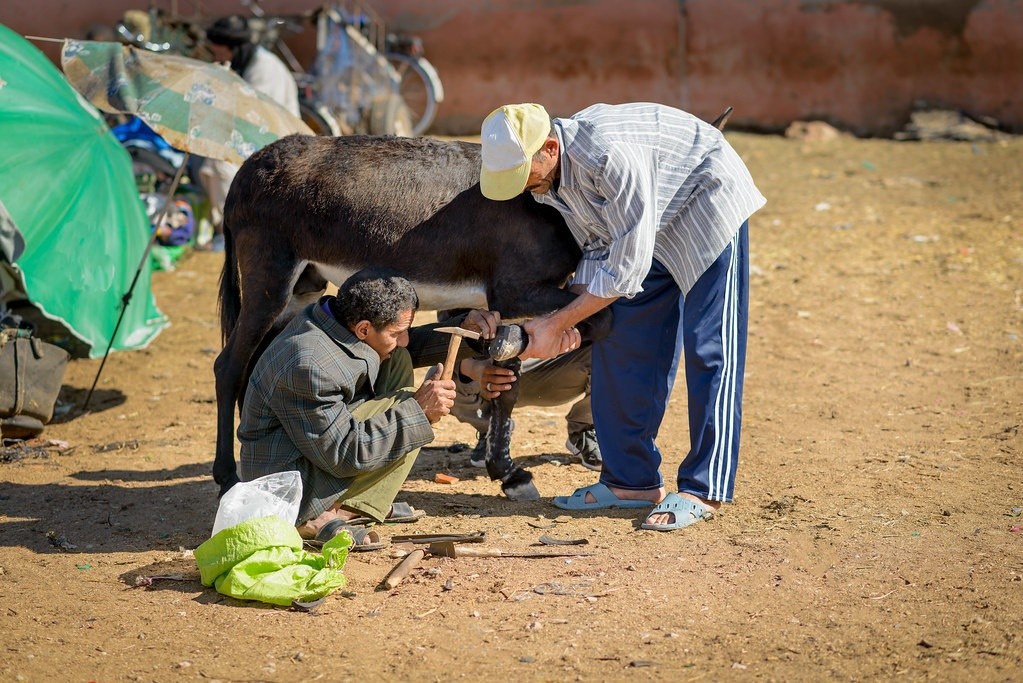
[0,338,70,424]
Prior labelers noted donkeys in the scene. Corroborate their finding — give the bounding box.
[206,106,734,500]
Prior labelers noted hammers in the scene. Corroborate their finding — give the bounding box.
[433,326,480,381]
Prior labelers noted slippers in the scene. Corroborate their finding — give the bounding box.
[641,491,718,530]
[302,518,387,550]
[346,502,425,524]
[552,483,653,510]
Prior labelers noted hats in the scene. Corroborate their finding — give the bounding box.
[481,103,550,200]
[206,15,251,45]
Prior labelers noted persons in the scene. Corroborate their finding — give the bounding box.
[236,265,500,552]
[426,307,602,472]
[197,14,301,251]
[479,103,768,530]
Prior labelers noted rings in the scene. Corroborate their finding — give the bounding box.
[487,383,491,391]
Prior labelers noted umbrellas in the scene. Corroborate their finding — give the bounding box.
[0,23,171,358]
[61,38,316,408]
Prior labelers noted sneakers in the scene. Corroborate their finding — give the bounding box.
[470,418,514,467]
[565,427,602,471]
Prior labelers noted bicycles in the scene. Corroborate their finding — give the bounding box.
[109,0,445,137]
